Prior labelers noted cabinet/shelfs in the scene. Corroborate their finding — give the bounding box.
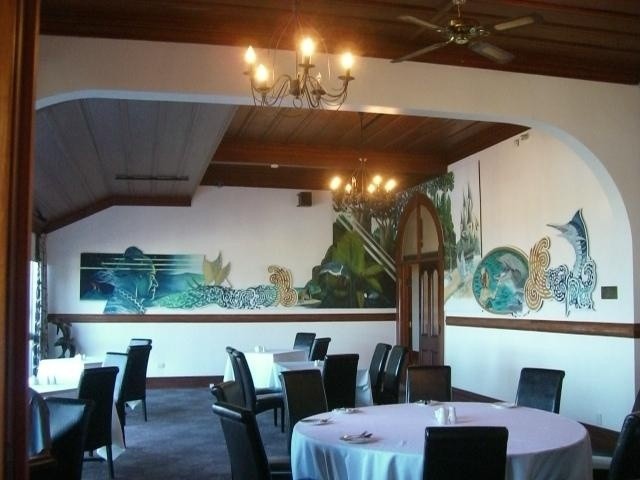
[31,376,57,386]
[436,406,457,426]
[314,360,320,367]
[255,344,265,353]
[78,352,86,361]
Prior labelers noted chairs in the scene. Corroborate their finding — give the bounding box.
[28,338,151,480]
[211,333,639,480]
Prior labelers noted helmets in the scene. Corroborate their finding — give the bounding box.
[413,400,439,407]
[332,408,358,414]
[338,433,375,443]
[491,402,516,408]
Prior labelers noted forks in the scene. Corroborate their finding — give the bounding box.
[340,431,372,441]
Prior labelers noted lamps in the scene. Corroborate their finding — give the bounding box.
[327,112,400,215]
[240,0,357,121]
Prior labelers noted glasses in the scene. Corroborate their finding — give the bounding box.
[240,0,357,121]
[327,112,400,215]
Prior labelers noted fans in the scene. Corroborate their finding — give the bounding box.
[387,0,543,65]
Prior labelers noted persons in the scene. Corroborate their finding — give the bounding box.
[479,263,526,310]
[102,246,158,314]
[81,275,104,300]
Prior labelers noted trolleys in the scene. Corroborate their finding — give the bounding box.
[387,0,543,65]
